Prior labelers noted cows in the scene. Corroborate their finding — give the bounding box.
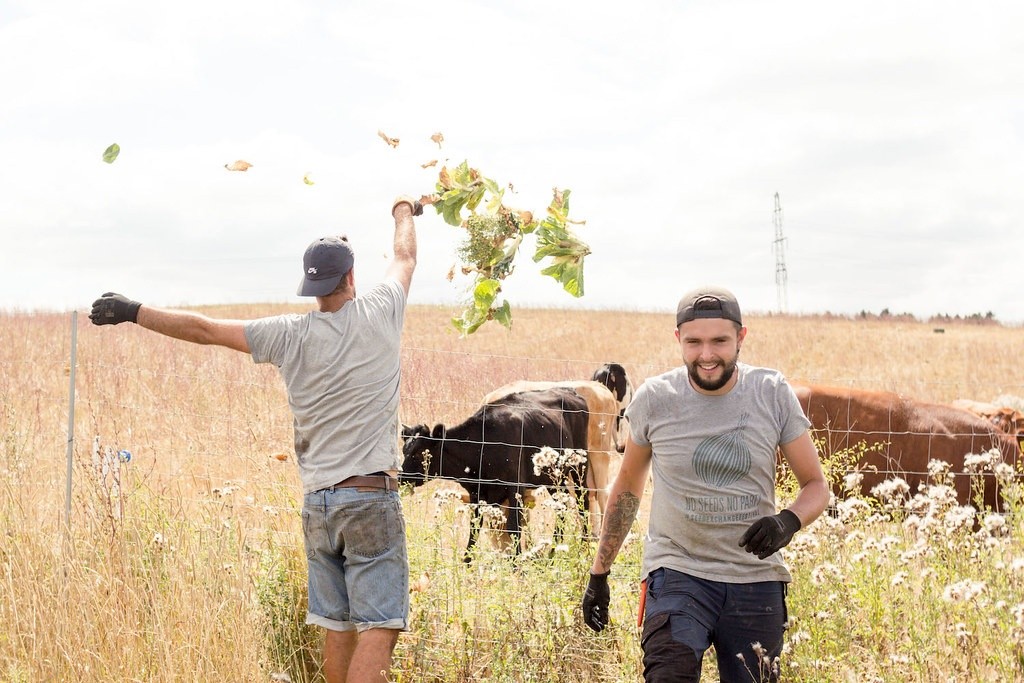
[775,382,1024,538]
[395,363,635,567]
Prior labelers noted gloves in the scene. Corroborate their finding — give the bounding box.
[88,292,143,326]
[739,508,801,559]
[392,199,423,217]
[583,569,612,632]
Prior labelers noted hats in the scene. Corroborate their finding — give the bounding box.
[298,237,355,296]
[676,286,742,329]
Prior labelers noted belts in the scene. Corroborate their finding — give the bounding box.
[325,475,399,492]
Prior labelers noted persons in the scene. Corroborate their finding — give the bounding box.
[583,288,831,683]
[89,194,425,683]
